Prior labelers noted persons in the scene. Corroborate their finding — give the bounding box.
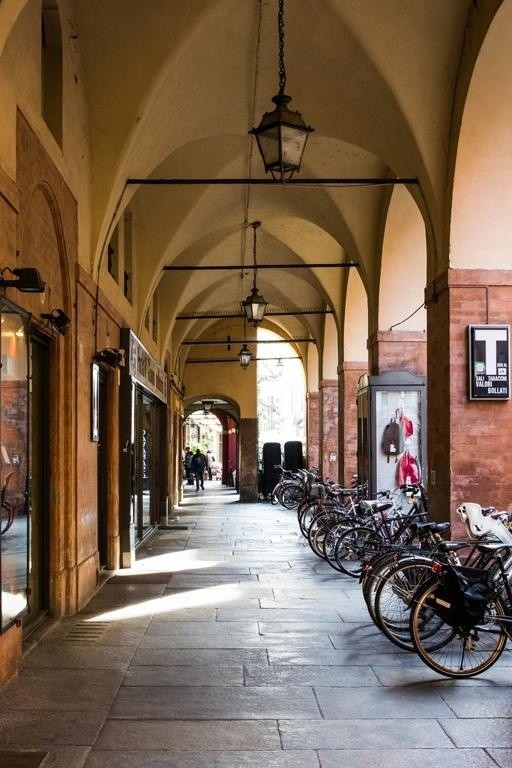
[183,447,215,490]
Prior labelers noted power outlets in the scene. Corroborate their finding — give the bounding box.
[1,260,47,310]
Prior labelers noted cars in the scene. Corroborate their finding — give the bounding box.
[210,461,222,475]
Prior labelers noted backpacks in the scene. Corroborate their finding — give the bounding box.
[381,417,400,455]
[192,454,204,470]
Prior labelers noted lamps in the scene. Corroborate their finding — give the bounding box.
[95,346,122,370]
[40,306,72,336]
[242,220,270,330]
[250,0,316,187]
[237,320,252,369]
